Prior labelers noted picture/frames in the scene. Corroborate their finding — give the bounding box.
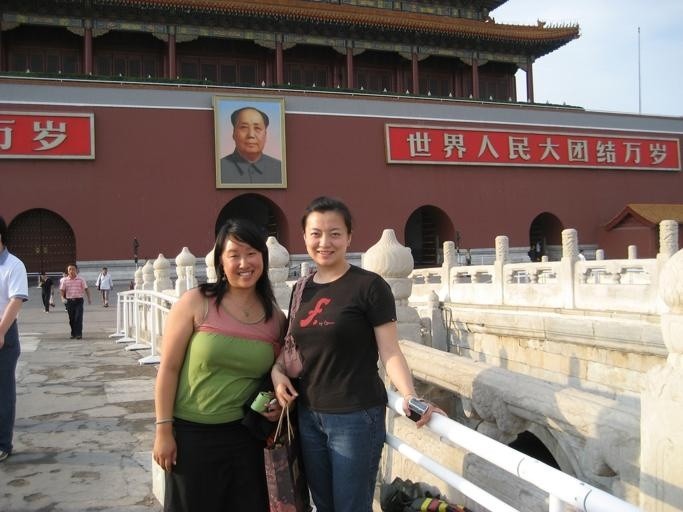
[212,93,288,188]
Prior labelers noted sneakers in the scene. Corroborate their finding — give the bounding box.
[0,450,9,461]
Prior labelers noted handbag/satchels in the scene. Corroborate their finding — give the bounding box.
[263,403,315,512]
[283,271,317,379]
[148,454,175,509]
[251,390,275,412]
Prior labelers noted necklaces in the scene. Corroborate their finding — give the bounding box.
[228,294,258,319]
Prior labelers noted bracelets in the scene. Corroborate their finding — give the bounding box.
[274,361,284,370]
[156,419,173,425]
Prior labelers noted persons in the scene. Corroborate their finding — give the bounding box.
[220,106,282,184]
[154,220,287,512]
[528,239,544,262]
[270,197,448,512]
[578,250,586,261]
[0,215,29,461]
[94,267,113,307]
[38,264,91,340]
[465,248,471,277]
